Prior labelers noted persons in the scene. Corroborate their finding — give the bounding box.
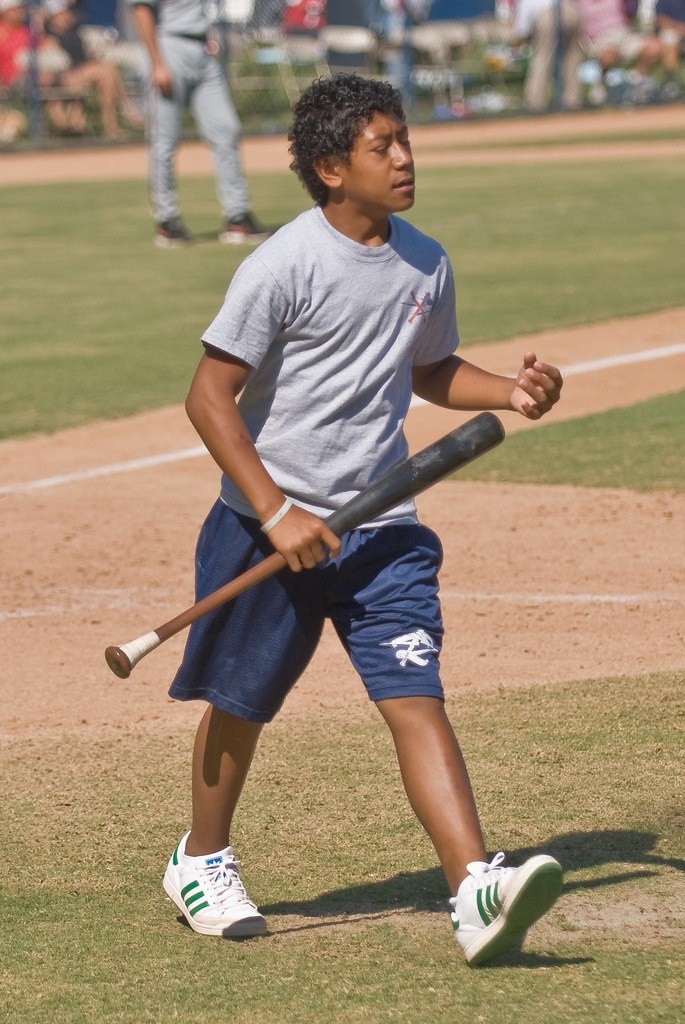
[280,0,474,120]
[0,0,147,143]
[164,76,563,965]
[493,0,685,114]
[131,0,272,247]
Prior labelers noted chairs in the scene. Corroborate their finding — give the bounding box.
[318,17,528,122]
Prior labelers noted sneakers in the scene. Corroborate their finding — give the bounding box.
[163,830,267,936]
[451,851,564,968]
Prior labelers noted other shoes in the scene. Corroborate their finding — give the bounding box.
[225,214,273,243]
[156,221,195,245]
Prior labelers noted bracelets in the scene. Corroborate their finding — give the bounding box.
[261,498,293,533]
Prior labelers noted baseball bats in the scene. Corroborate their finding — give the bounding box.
[104,411,507,680]
